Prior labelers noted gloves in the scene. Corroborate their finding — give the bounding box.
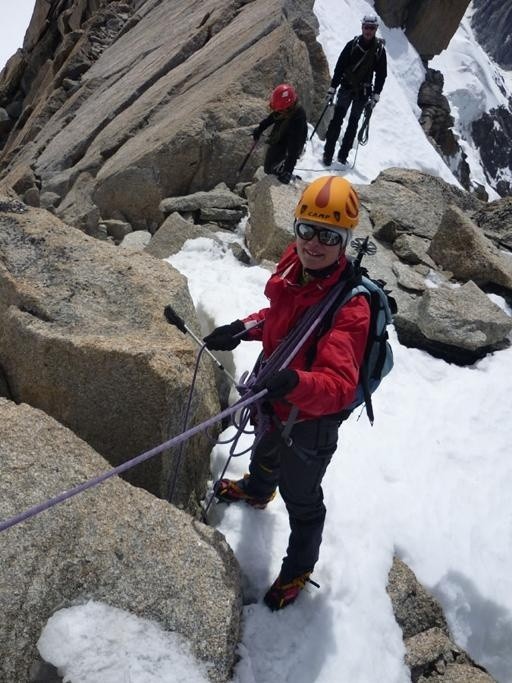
[278,167,292,184]
[252,126,262,142]
[253,367,297,400]
[369,93,380,107]
[203,320,246,351]
[324,87,336,104]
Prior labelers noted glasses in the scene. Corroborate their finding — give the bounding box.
[295,222,343,246]
[363,25,377,30]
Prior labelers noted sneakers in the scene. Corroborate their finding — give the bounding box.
[213,475,277,510]
[323,152,348,166]
[264,567,311,610]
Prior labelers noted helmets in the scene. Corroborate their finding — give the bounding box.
[361,14,379,25]
[294,175,360,228]
[270,84,296,111]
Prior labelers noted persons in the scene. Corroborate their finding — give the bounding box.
[322,12,388,168]
[252,83,307,183]
[200,176,376,613]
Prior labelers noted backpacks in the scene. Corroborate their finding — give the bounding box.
[315,263,398,422]
